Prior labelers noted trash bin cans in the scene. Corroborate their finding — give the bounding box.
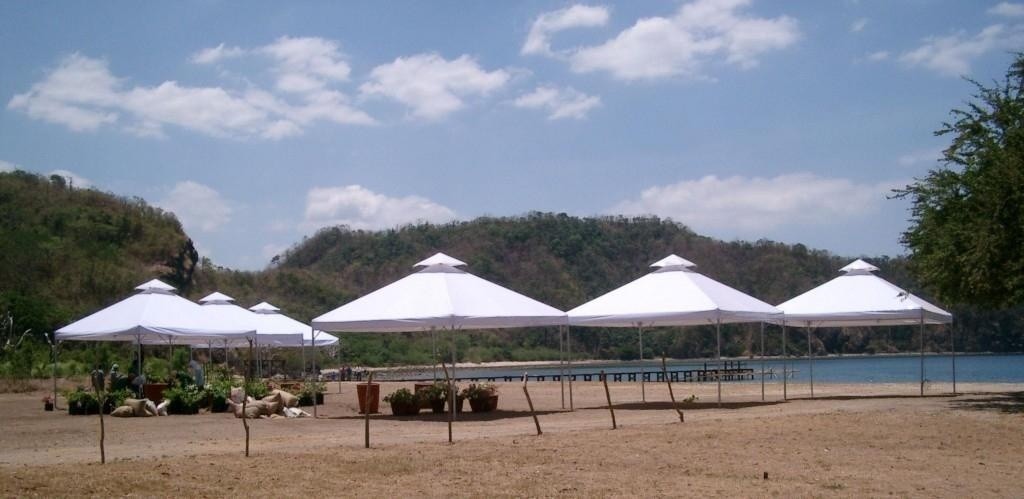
[356,383,380,413]
[414,383,434,395]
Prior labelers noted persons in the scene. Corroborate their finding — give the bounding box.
[126,360,142,398]
[339,365,346,381]
[345,364,352,381]
[89,363,104,394]
[355,365,361,381]
[109,364,123,391]
[188,359,204,391]
[174,373,191,388]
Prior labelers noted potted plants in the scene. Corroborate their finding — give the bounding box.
[465,380,498,412]
[45,395,57,412]
[446,382,464,413]
[69,362,327,414]
[427,382,444,411]
[385,388,420,413]
